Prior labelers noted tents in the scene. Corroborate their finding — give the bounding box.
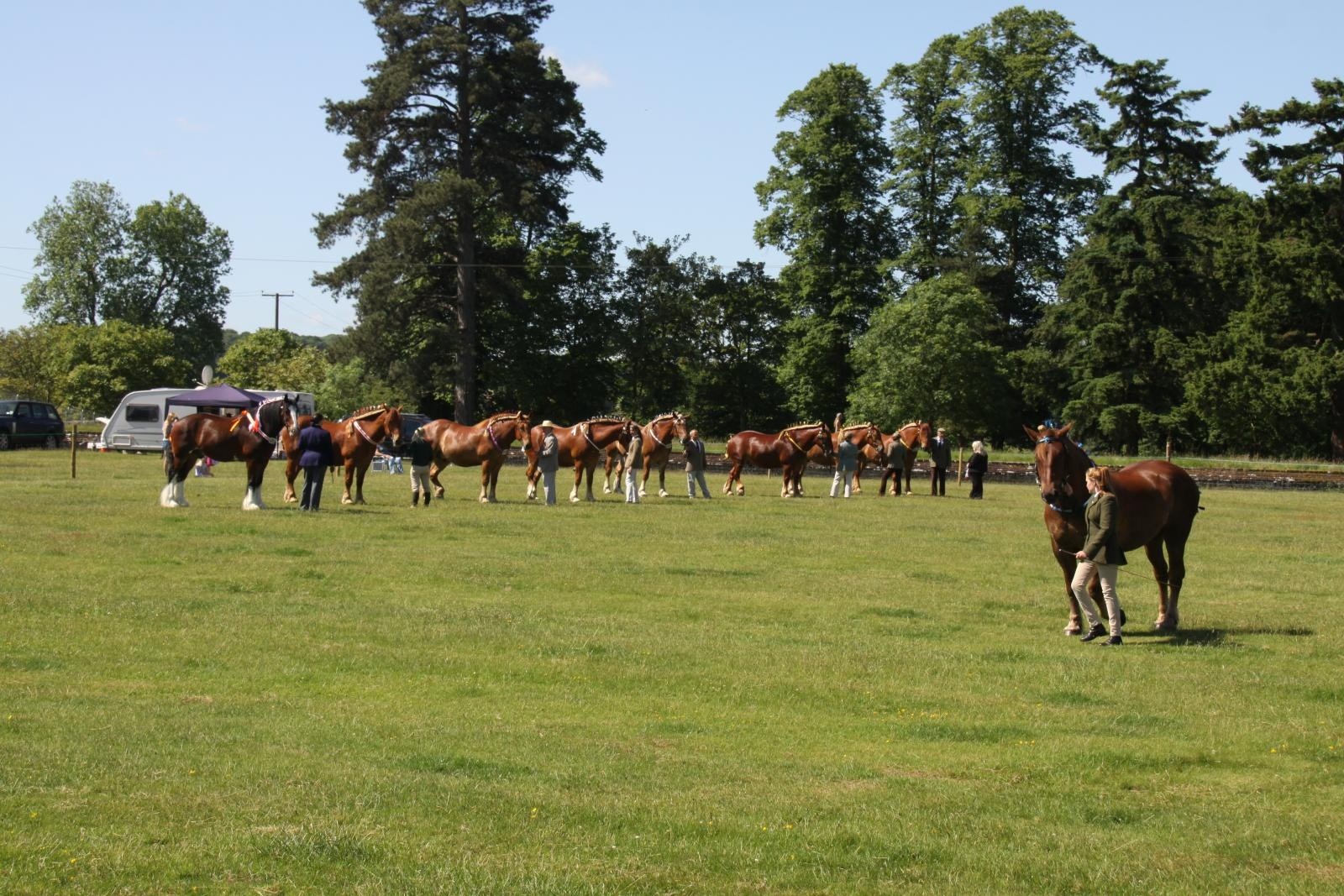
[164,384,268,420]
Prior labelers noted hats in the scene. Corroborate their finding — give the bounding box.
[938,428,945,432]
[538,420,554,429]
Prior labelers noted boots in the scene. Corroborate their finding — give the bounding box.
[409,491,421,508]
[424,492,431,507]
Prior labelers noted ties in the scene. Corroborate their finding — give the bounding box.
[696,441,699,452]
[938,439,941,448]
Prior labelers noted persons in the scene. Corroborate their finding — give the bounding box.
[162,413,180,474]
[682,430,712,498]
[536,420,559,504]
[965,439,989,497]
[928,428,952,497]
[392,427,435,508]
[878,432,904,497]
[1071,465,1125,649]
[831,433,859,499]
[624,426,642,502]
[294,412,334,512]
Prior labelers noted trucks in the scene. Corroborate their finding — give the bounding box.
[86,364,316,459]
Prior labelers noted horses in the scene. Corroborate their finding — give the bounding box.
[1021,423,1200,641]
[417,412,691,505]
[722,419,931,497]
[159,394,404,511]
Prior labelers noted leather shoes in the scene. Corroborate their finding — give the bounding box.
[1080,623,1107,641]
[1099,632,1124,646]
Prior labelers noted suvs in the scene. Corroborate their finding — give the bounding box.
[0,399,67,452]
[336,410,433,458]
